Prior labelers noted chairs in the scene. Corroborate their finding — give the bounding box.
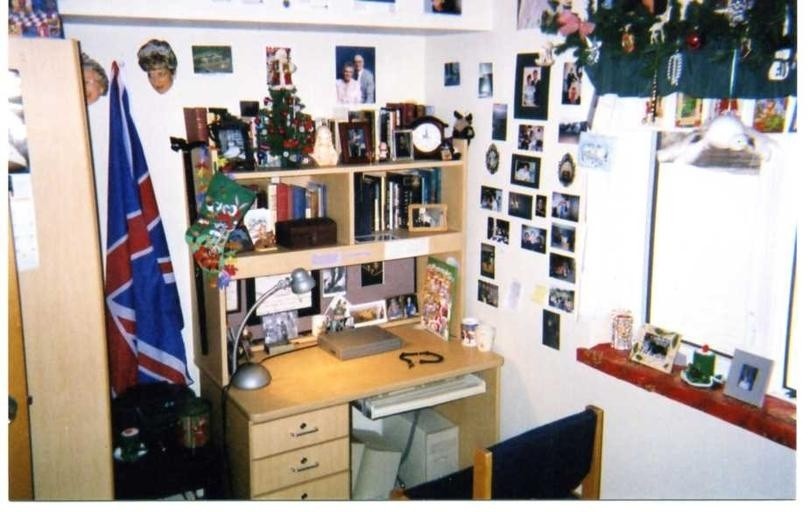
[384,401,605,500]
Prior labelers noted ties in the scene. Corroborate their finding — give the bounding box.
[354,71,358,80]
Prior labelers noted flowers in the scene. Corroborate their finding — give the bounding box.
[539,0,604,69]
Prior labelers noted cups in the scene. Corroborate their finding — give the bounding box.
[612,314,634,351]
[460,318,480,348]
[476,324,494,351]
[120,427,140,462]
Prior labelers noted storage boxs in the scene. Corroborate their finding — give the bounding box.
[272,215,338,250]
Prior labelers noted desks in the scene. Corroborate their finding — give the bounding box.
[169,320,508,498]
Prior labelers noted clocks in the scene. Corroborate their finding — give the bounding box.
[409,115,449,161]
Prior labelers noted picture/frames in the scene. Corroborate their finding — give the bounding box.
[333,118,375,165]
[208,111,254,170]
[245,269,323,328]
[509,152,542,189]
[720,347,776,410]
[630,320,682,374]
[509,49,552,122]
[406,203,451,234]
[225,277,244,315]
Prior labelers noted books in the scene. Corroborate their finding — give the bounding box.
[346,101,430,163]
[245,177,328,224]
[180,103,281,169]
[354,167,445,242]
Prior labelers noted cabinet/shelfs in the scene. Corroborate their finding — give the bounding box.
[173,104,475,392]
[112,382,231,501]
[9,30,124,499]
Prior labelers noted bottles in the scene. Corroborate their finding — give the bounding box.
[332,308,346,333]
[693,344,716,376]
[388,296,416,319]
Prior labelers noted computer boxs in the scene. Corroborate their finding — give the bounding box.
[380,408,461,485]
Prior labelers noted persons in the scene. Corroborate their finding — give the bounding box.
[352,54,374,102]
[80,50,109,105]
[476,64,582,314]
[336,64,363,102]
[138,40,179,95]
[387,294,416,321]
[738,376,751,390]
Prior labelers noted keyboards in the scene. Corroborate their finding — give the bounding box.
[364,374,472,407]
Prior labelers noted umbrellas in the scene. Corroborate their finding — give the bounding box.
[103,62,194,396]
